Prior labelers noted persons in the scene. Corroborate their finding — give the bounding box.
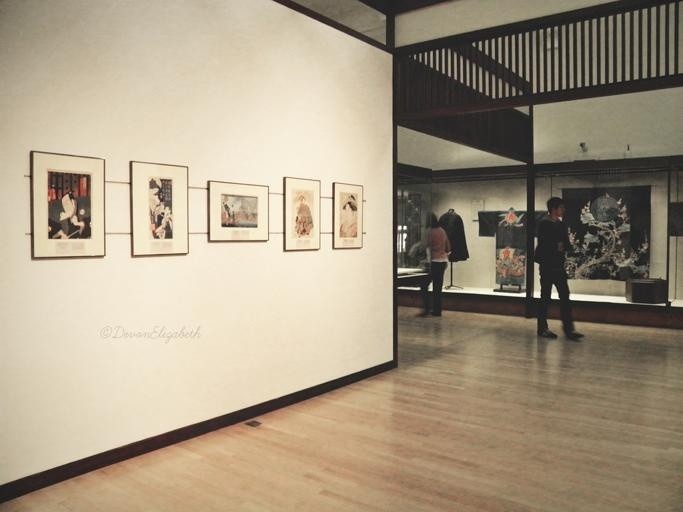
[535,197,584,338]
[416,212,451,316]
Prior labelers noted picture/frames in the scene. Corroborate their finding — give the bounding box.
[28,149,106,259]
[331,181,364,251]
[206,179,269,243]
[282,176,322,253]
[128,160,190,257]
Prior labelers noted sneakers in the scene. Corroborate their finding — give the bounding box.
[538,330,586,339]
[416,311,441,317]
